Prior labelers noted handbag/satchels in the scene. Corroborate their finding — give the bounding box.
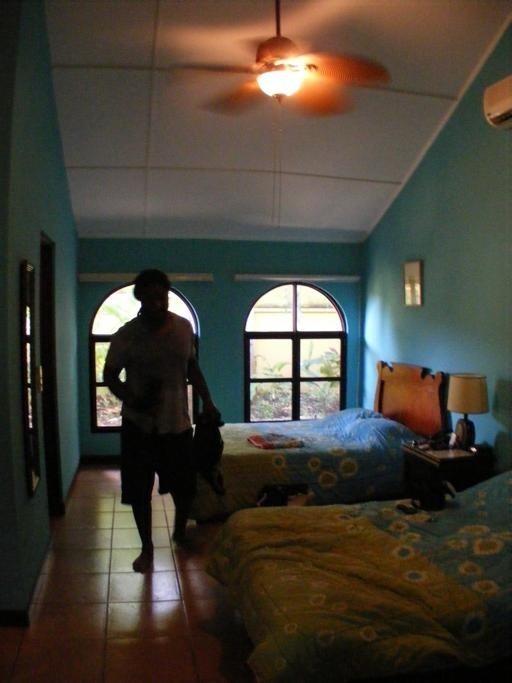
[192,407,224,470]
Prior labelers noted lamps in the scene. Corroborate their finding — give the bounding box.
[254,71,304,101]
[447,374,490,450]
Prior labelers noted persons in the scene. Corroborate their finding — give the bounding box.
[102,268,215,572]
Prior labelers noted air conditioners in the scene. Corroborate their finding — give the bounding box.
[484,73,511,128]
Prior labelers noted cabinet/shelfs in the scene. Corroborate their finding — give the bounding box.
[402,440,490,501]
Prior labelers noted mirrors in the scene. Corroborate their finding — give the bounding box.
[21,262,40,500]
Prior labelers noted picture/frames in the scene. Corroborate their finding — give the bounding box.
[402,260,423,308]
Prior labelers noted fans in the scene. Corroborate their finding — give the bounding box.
[165,1,390,118]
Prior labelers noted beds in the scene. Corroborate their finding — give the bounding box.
[183,362,447,519]
[216,467,510,682]
